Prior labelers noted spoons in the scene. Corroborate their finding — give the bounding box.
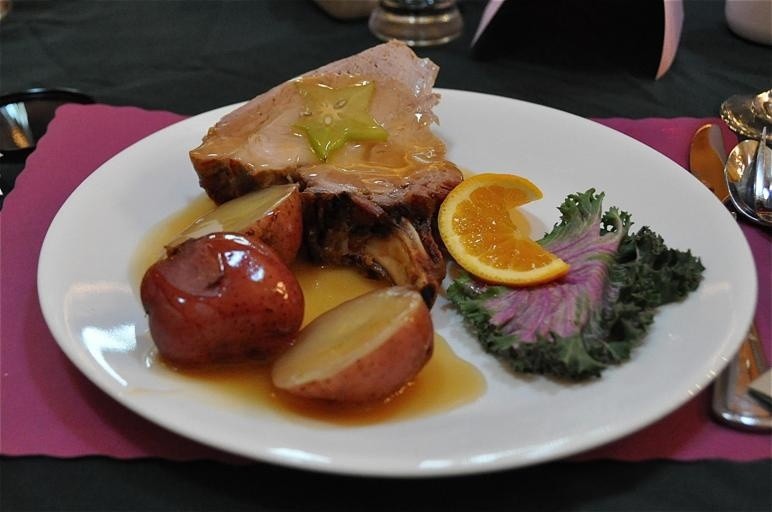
[751,89,771,226]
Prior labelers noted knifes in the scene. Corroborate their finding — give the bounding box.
[686,124,772,428]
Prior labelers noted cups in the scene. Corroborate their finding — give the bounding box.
[368,0,463,47]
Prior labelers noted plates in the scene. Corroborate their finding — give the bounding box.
[38,84,758,479]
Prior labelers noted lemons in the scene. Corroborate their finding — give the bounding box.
[439,172,570,286]
